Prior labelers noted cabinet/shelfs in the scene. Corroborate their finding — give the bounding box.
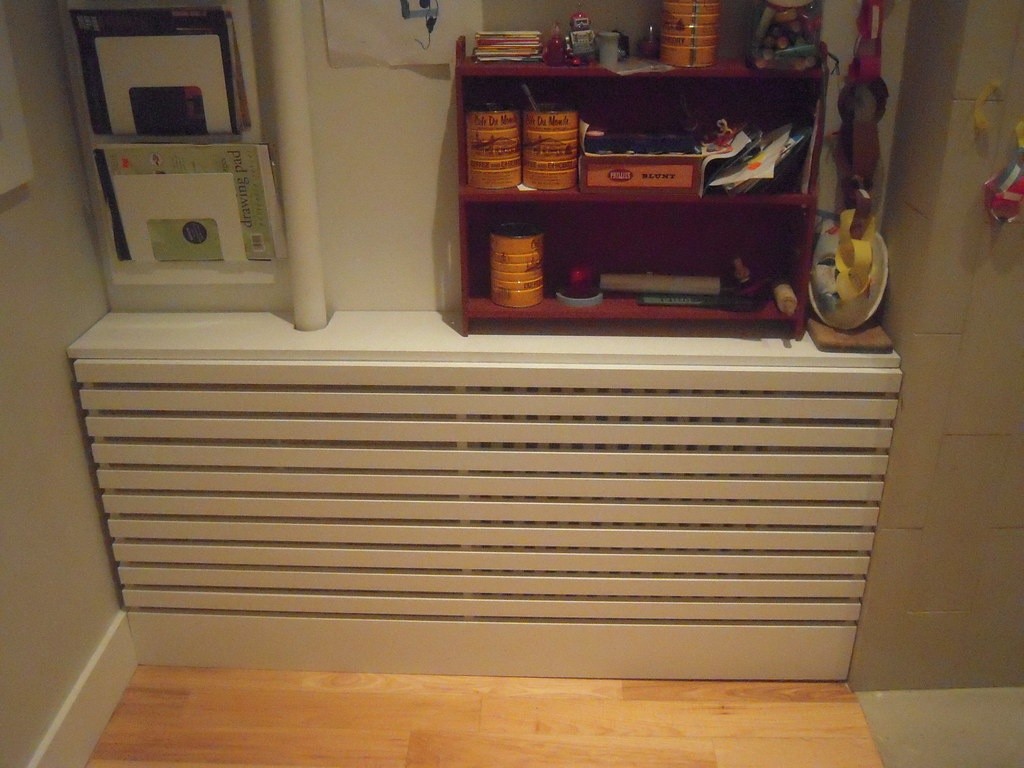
[450,36,833,340]
